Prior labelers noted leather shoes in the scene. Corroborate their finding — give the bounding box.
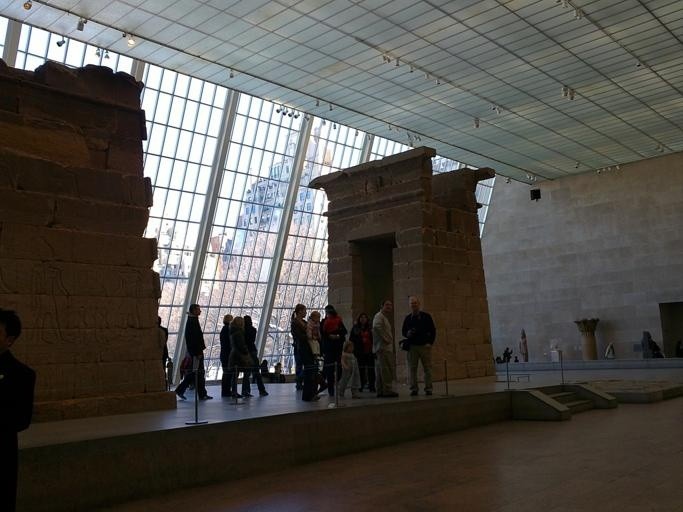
[176,390,268,401]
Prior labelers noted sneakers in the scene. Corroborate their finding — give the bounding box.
[312,386,432,402]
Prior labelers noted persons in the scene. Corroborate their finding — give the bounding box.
[639,330,663,358]
[1,308,38,511]
[156,294,437,402]
